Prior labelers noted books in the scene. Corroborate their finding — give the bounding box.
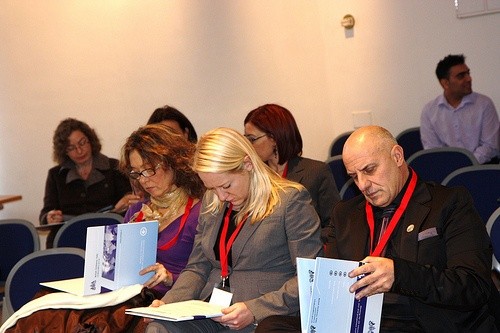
[124,300,226,322]
[296,256,384,333]
[39,220,159,297]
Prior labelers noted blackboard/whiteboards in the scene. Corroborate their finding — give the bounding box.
[455,0,500,18]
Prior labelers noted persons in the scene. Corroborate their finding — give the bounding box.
[243,104,342,229]
[254,126,493,333]
[420,55,500,165]
[39,117,141,249]
[143,128,325,333]
[147,105,198,145]
[4,124,205,333]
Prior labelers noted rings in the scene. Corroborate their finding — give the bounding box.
[156,272,160,277]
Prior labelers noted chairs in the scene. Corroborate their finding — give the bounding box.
[320,124,500,296]
[0,212,126,316]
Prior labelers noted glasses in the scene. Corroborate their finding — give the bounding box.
[65,140,90,153]
[129,160,163,179]
[244,134,268,144]
